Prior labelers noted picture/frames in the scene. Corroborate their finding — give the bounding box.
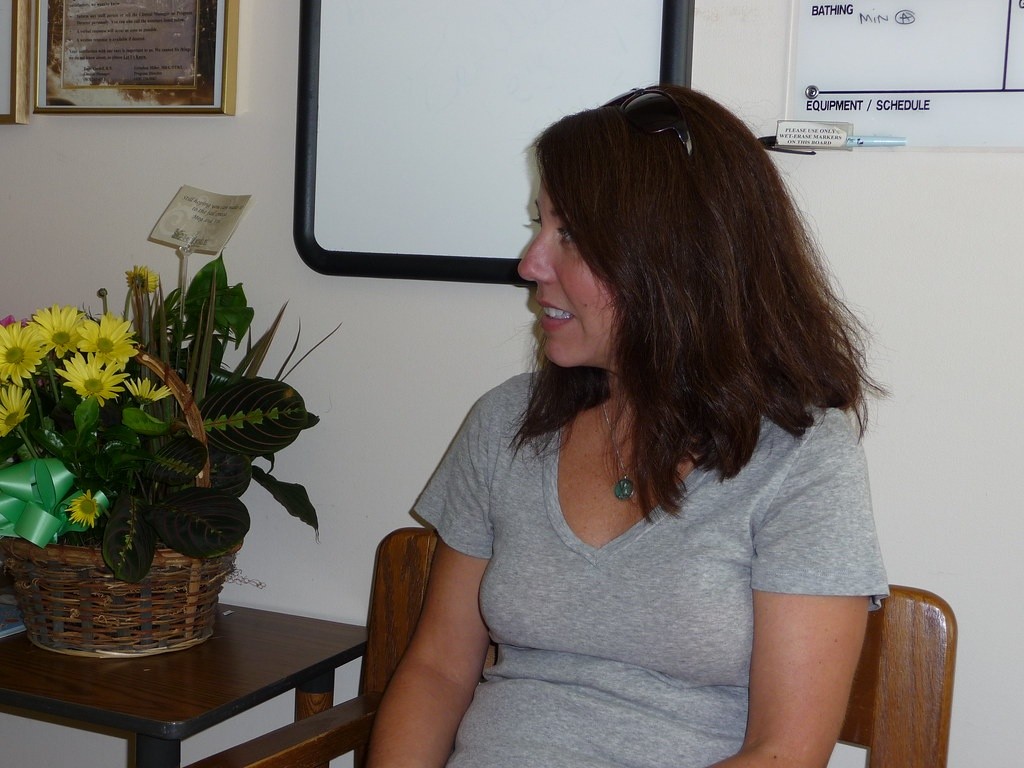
[1,0,30,126]
[32,0,241,117]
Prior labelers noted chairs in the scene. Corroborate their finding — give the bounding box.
[186,526,961,768]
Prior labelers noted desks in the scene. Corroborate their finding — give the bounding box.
[0,586,368,768]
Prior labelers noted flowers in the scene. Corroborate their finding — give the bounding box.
[1,183,322,584]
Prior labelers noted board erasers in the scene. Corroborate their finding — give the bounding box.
[773,119,855,152]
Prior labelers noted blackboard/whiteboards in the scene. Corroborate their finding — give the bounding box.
[773,0,1024,154]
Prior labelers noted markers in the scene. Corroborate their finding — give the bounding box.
[845,135,907,147]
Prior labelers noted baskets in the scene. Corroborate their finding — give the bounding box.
[1,343,243,659]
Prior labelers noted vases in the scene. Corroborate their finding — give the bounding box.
[0,349,246,659]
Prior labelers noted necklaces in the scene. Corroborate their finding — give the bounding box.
[603,405,637,501]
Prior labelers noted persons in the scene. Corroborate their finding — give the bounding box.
[364,85,891,767]
